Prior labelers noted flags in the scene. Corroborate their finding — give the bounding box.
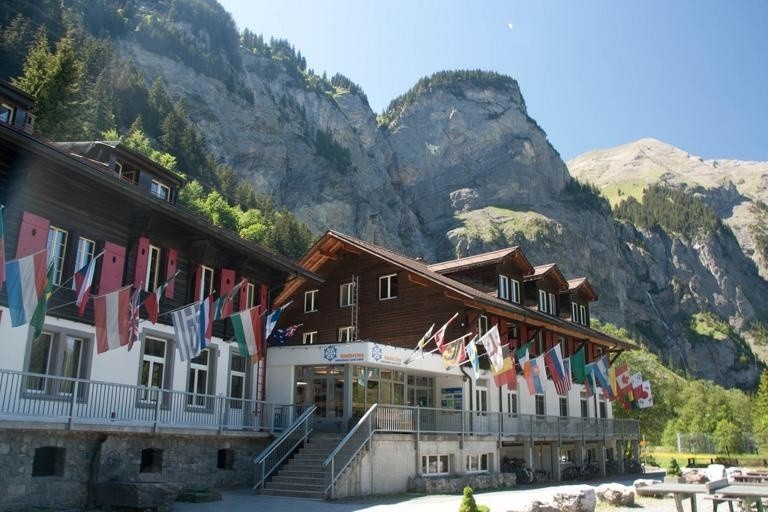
[4,249,299,361]
[417,320,654,411]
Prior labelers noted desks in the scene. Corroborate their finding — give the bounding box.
[637,471,768,512]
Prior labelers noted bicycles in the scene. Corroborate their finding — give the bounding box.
[498,455,538,488]
[558,454,648,481]
